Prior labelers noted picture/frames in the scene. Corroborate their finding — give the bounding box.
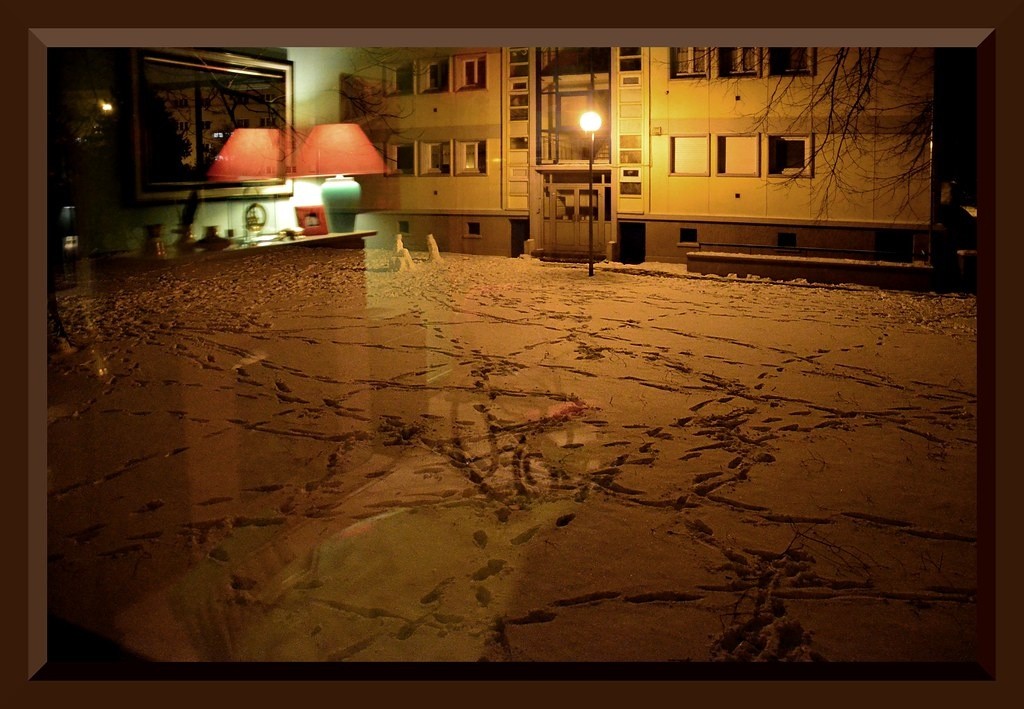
[121,46,295,205]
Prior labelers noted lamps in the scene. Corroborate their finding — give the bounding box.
[290,121,392,233]
[209,128,294,239]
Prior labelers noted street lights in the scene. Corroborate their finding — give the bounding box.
[581,110,602,274]
